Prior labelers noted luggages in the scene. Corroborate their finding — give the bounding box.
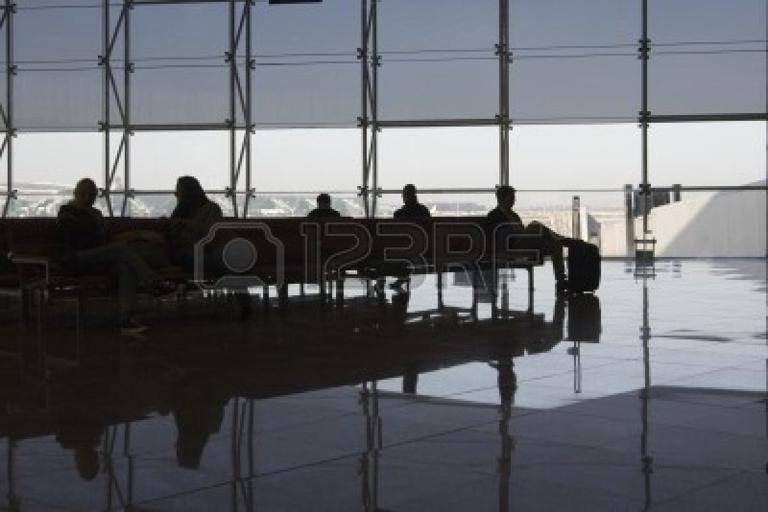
[567,238,602,292]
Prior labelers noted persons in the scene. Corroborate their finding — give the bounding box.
[373,184,432,294]
[496,293,566,401]
[487,185,587,295]
[303,190,344,219]
[159,175,226,273]
[159,400,234,471]
[46,175,178,337]
[58,418,110,483]
